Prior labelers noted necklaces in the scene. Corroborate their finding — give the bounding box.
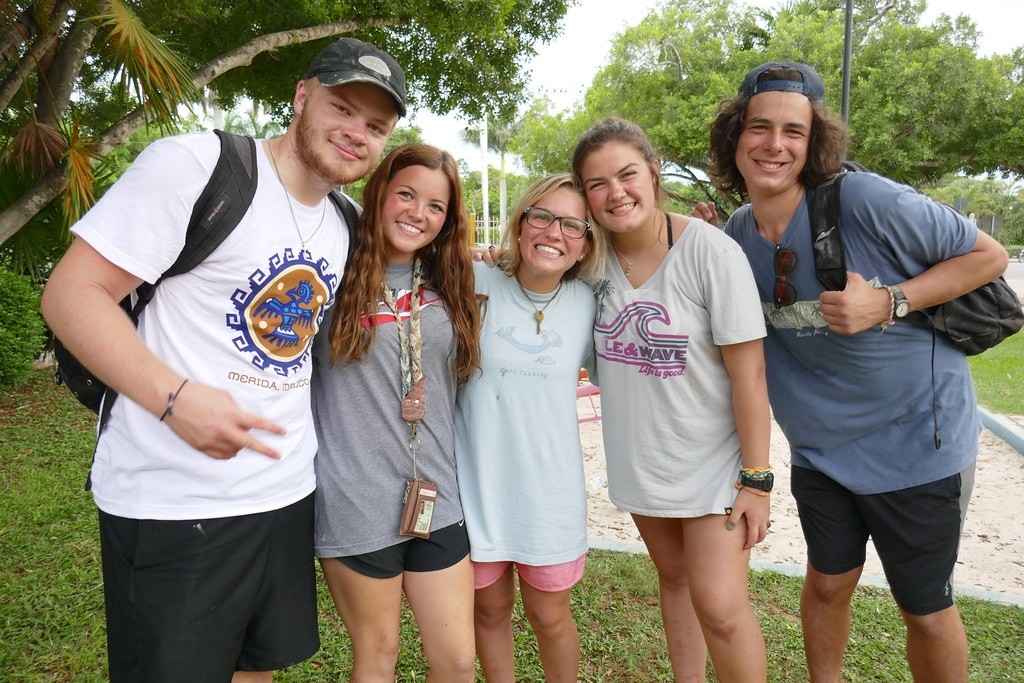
[515,271,562,312]
[268,140,327,261]
[612,239,659,278]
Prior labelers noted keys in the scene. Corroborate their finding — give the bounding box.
[535,311,545,335]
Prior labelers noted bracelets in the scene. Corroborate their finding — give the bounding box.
[738,465,773,479]
[734,481,773,497]
[877,284,895,335]
[160,378,188,421]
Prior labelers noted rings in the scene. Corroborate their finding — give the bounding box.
[488,245,495,249]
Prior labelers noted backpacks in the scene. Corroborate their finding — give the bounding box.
[809,158,1024,356]
[54,128,360,415]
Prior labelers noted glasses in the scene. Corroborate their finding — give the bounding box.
[525,208,591,239]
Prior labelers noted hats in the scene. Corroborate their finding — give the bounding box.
[740,62,825,103]
[303,38,408,119]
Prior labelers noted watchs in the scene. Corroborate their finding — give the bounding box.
[888,286,911,318]
[739,471,774,492]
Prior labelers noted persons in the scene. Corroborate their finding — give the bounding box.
[420,501,424,514]
[684,60,1010,683]
[471,118,774,683]
[40,36,408,683]
[464,171,605,683]
[310,143,483,683]
